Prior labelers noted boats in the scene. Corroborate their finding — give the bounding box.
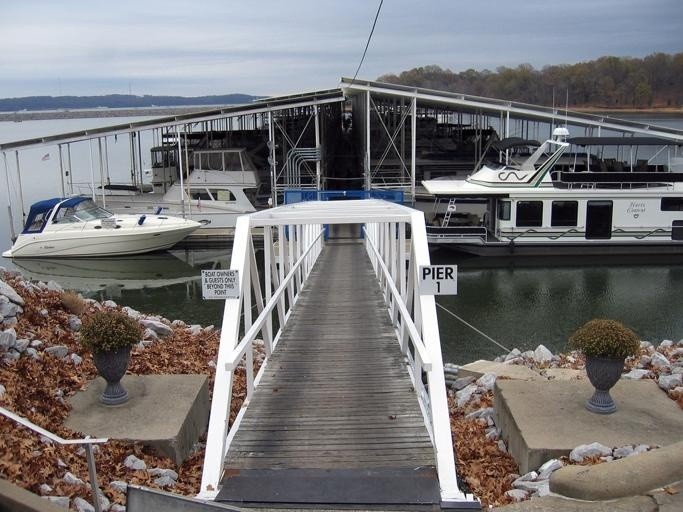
[2,130,279,259]
[428,245,683,306]
[12,248,257,291]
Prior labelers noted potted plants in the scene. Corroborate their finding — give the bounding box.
[570,319,644,415]
[74,298,145,404]
